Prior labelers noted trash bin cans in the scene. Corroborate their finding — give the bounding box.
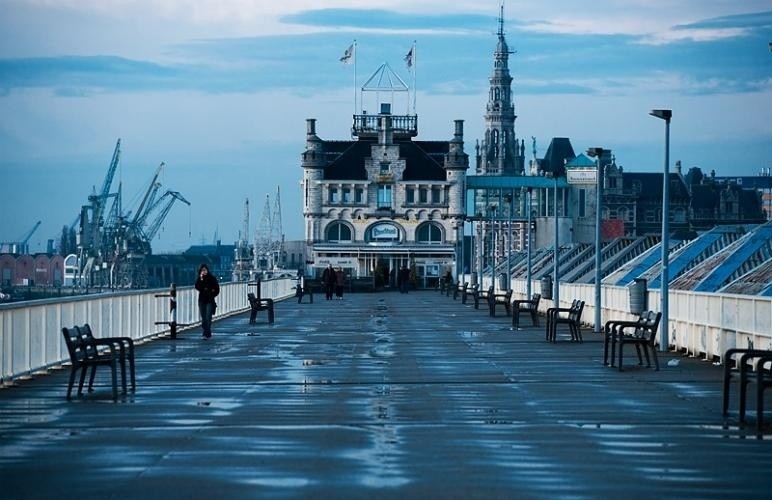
[499,273,507,290]
[629,278,646,315]
[472,272,477,285]
[540,274,552,299]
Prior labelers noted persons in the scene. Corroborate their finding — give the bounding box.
[442,268,453,292]
[400,266,411,294]
[320,263,337,300]
[388,265,396,286]
[335,266,347,300]
[194,263,221,340]
[397,266,403,287]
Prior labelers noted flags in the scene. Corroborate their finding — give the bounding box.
[338,42,354,64]
[403,47,416,69]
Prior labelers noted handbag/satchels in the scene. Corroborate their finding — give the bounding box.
[211,301,217,315]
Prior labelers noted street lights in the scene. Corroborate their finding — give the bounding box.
[520,185,531,308]
[649,109,672,352]
[584,147,604,333]
[502,194,512,290]
[543,169,559,318]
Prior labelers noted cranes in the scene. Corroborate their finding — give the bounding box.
[61,137,191,254]
[19,220,42,244]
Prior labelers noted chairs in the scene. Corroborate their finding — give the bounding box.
[248,292,275,323]
[440,281,513,318]
[512,292,541,328]
[546,298,585,344]
[603,310,662,370]
[61,323,136,400]
